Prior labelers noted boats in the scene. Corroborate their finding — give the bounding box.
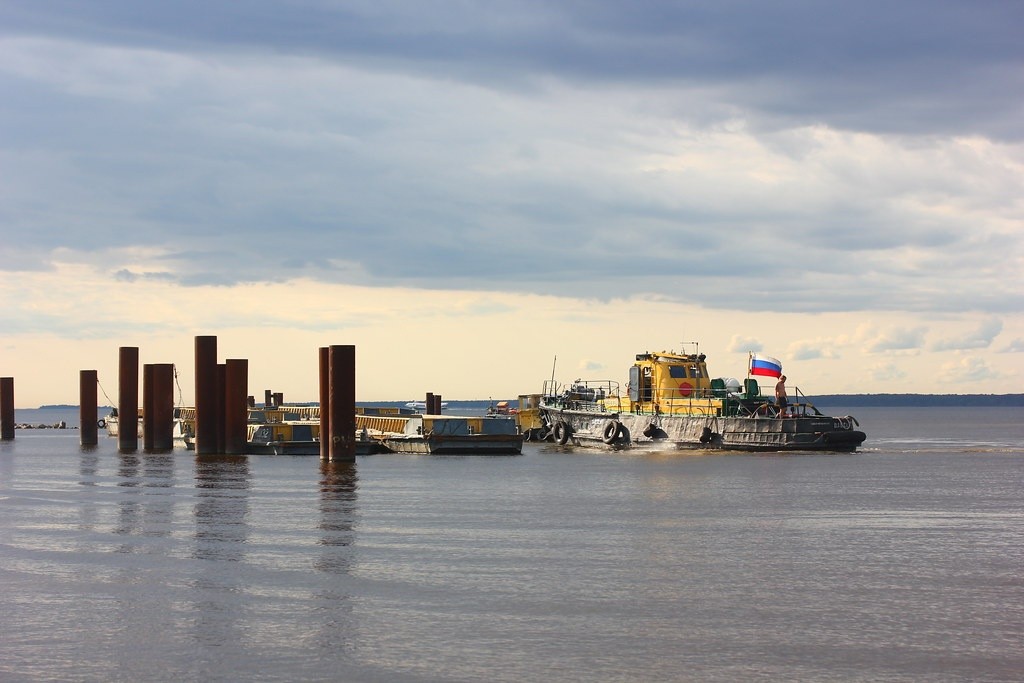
[539,343,866,453]
[102,414,188,439]
[178,389,524,456]
[485,392,544,441]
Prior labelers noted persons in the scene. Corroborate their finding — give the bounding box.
[774,375,790,419]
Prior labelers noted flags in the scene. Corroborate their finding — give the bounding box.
[750,353,782,380]
